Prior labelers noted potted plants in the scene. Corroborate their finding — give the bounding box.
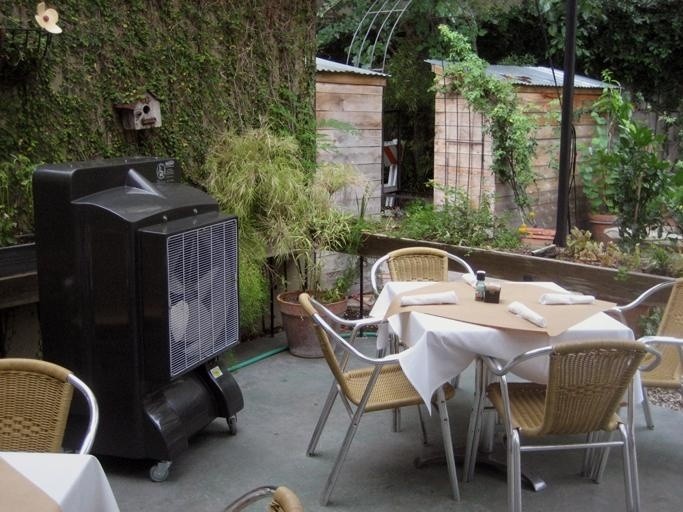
[205,123,348,358]
[434,22,556,251]
[588,69,627,242]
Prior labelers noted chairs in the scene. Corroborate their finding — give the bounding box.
[583,276,682,477]
[463,342,662,512]
[223,487,304,512]
[371,246,476,432]
[0,358,100,453]
[298,293,461,507]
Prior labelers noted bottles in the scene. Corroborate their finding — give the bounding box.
[474,271,486,300]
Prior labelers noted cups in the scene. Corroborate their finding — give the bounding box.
[484,285,502,303]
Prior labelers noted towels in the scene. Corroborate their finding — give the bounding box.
[540,292,597,306]
[462,273,478,287]
[400,291,456,307]
[507,301,548,328]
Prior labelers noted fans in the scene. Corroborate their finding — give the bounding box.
[32,157,243,483]
